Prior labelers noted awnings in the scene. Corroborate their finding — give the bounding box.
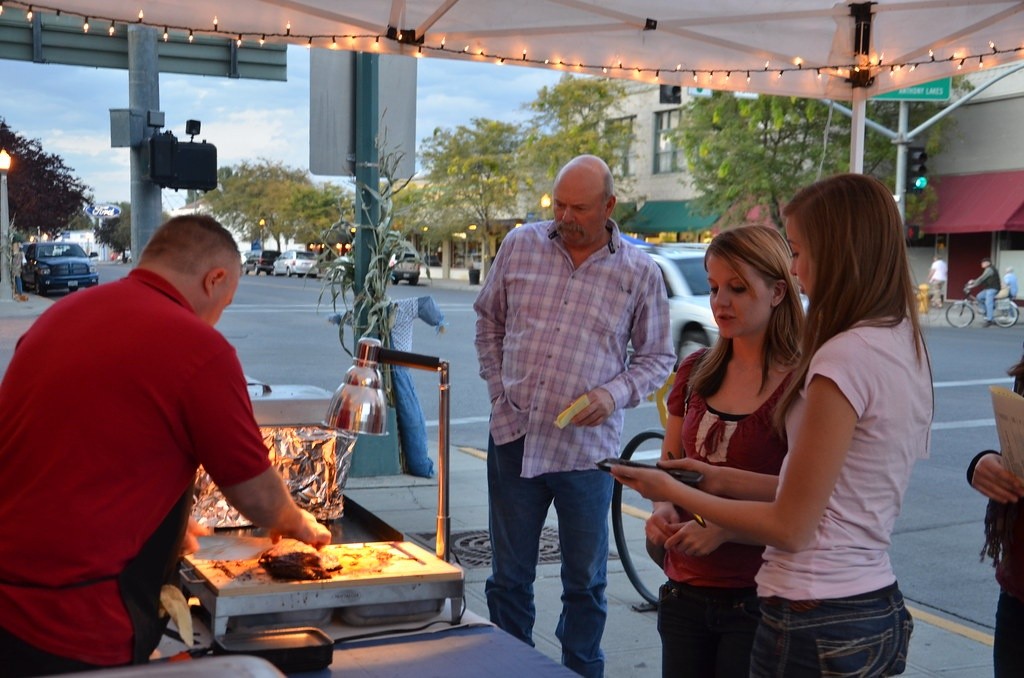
[711,189,789,234]
[609,201,637,225]
[907,170,1024,234]
[622,198,736,233]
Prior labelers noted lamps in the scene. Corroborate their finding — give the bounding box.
[321,338,453,562]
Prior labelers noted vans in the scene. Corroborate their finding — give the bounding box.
[389,251,421,286]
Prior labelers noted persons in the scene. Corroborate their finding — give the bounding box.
[0,212,333,678]
[645,226,806,678]
[968,258,1001,323]
[967,343,1024,678]
[612,173,936,678]
[1003,266,1017,319]
[472,154,678,678]
[929,254,947,308]
[328,297,448,479]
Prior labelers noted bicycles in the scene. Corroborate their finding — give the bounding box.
[944,289,1020,329]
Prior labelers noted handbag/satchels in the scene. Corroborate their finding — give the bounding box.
[645,345,712,571]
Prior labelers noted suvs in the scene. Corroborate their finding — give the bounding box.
[627,241,811,375]
[242,250,282,276]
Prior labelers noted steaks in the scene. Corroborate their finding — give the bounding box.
[259,538,323,582]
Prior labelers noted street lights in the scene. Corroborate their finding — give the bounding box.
[0,149,11,300]
[540,193,551,222]
[259,218,265,250]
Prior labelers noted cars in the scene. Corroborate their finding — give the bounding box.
[21,242,98,297]
[325,255,352,280]
[272,250,319,278]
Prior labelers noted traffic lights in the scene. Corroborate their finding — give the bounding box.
[904,145,928,194]
[659,84,683,104]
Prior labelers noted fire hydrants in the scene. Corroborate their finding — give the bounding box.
[915,283,930,313]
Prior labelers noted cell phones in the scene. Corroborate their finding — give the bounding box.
[596,457,704,483]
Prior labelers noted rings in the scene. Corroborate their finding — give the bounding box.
[649,535,655,542]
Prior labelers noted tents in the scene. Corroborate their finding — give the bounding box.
[1,0,1024,173]
